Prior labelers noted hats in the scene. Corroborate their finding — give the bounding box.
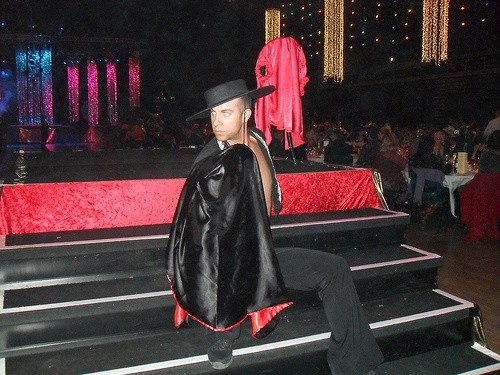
[186,79,275,122]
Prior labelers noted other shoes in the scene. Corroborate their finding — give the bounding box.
[208,332,233,370]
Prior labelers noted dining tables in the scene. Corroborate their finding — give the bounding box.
[441,172,476,220]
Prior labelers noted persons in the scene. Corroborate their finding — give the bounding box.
[0,117,8,152]
[167,77,385,375]
[40,115,48,151]
[116,101,500,218]
